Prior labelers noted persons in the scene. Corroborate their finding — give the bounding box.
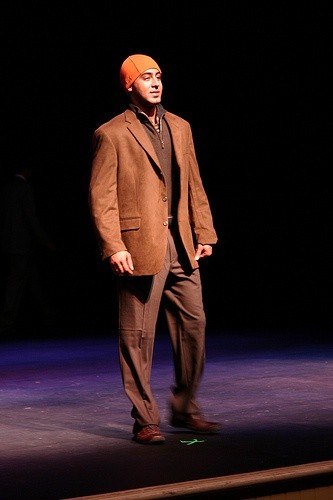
[91,53,224,444]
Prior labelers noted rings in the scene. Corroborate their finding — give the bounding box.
[114,269,118,273]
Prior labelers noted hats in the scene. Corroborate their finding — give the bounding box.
[120,54,162,93]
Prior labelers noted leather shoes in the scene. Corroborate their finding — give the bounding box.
[170,414,224,432]
[134,424,166,444]
